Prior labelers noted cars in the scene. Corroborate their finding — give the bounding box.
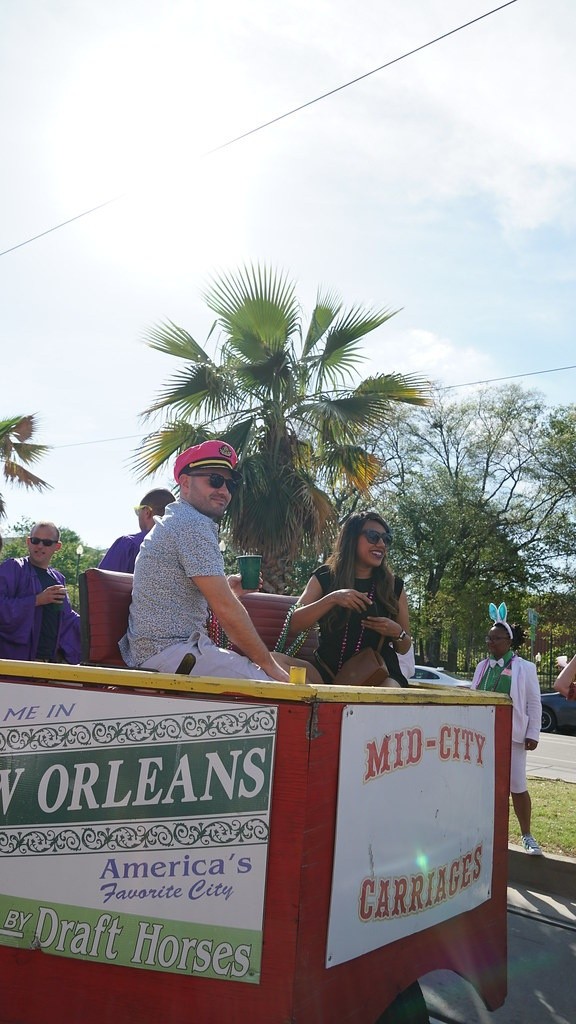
[540,692,576,737]
[409,664,472,689]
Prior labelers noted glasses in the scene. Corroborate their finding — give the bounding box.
[484,635,508,642]
[30,537,58,547]
[360,529,393,547]
[186,472,235,494]
[133,505,153,516]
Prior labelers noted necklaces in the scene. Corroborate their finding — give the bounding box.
[206,606,234,650]
[275,603,311,659]
[482,660,511,692]
[338,578,377,672]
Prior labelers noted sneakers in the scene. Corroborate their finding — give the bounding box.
[520,835,542,856]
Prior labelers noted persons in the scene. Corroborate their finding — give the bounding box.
[552,653,576,703]
[469,623,544,857]
[536,651,542,672]
[290,512,413,689]
[116,440,293,684]
[94,488,177,574]
[0,522,83,665]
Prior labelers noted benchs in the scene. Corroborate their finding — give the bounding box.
[79,568,319,670]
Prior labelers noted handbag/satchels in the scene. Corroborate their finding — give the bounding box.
[333,648,390,686]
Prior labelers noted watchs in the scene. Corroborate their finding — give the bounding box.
[396,630,406,642]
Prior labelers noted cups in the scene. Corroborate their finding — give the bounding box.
[236,555,263,590]
[52,588,67,608]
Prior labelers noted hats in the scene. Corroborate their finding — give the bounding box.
[174,440,237,483]
